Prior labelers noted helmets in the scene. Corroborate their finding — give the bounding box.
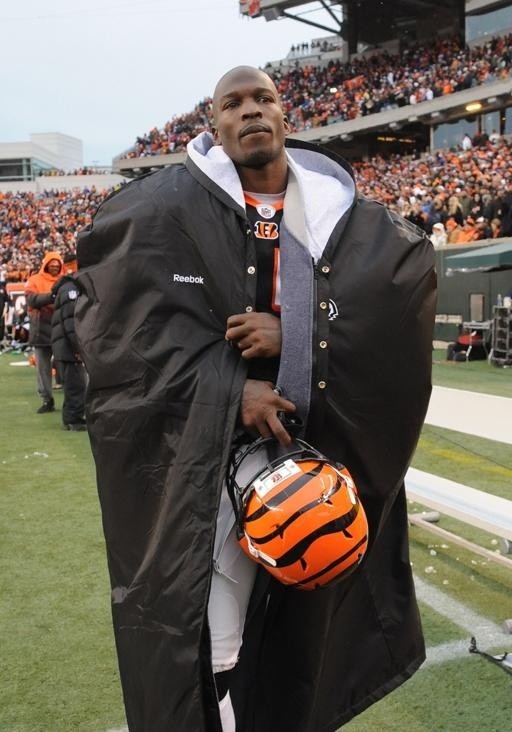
[235,450,369,594]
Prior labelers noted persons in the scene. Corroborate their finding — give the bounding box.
[125,97,215,158]
[39,167,94,175]
[349,131,510,245]
[52,271,89,432]
[74,67,438,732]
[261,34,512,134]
[1,184,124,353]
[25,253,69,413]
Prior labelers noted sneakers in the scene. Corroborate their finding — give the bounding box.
[37,398,55,413]
[63,419,86,431]
